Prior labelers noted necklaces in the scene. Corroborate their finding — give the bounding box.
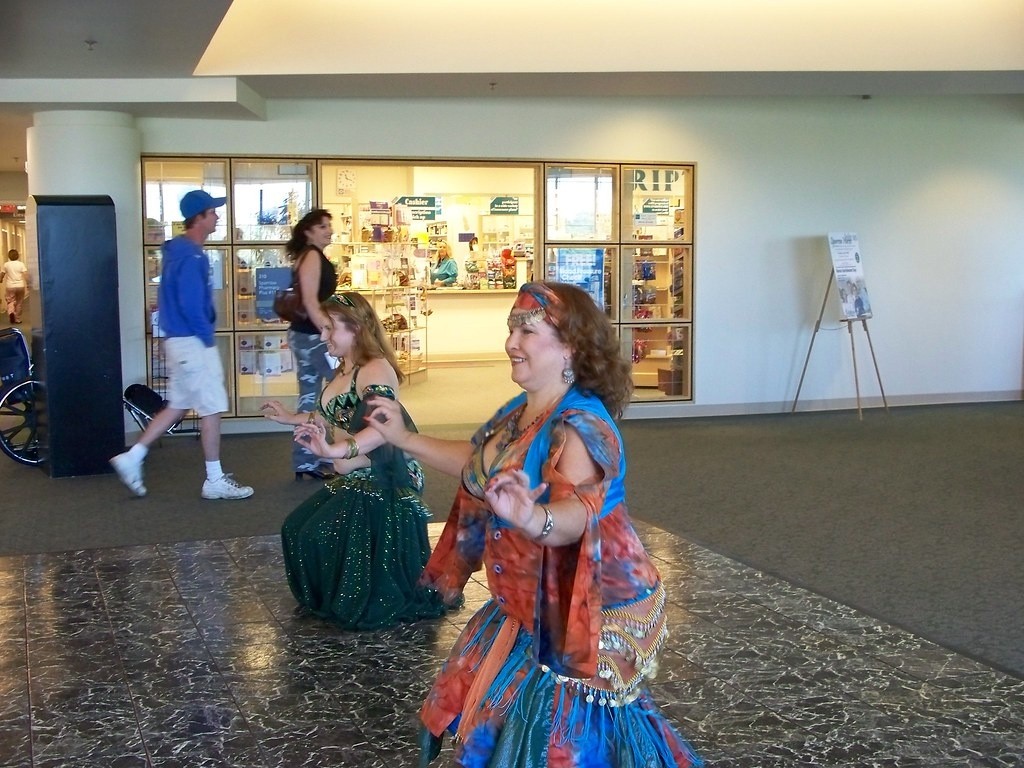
[340,363,356,377]
[496,402,546,453]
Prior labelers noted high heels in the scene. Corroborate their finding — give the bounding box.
[295,468,335,481]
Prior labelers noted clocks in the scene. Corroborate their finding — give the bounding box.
[336,168,360,196]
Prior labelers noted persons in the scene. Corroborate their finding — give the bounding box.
[430,240,458,288]
[840,281,872,318]
[272,209,339,481]
[363,281,706,768]
[0,249,29,324]
[110,190,254,500]
[469,237,478,251]
[256,292,466,624]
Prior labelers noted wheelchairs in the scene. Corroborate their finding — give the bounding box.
[0,326,48,467]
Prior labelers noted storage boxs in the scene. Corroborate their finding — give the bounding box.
[657,368,683,393]
[146,219,295,379]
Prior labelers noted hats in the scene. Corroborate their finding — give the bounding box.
[180,190,228,219]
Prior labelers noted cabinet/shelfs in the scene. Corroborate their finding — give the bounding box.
[428,220,454,259]
[322,201,395,286]
[478,214,535,260]
[330,287,428,385]
[631,254,672,388]
[669,206,684,368]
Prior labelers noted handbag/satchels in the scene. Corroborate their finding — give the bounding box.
[273,288,303,321]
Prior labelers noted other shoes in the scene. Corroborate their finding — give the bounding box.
[10,313,15,323]
[17,322,21,324]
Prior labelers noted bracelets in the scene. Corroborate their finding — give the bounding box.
[531,504,554,542]
[340,438,360,460]
[306,412,316,426]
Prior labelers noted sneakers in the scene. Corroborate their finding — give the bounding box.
[200,472,254,499]
[108,453,147,497]
